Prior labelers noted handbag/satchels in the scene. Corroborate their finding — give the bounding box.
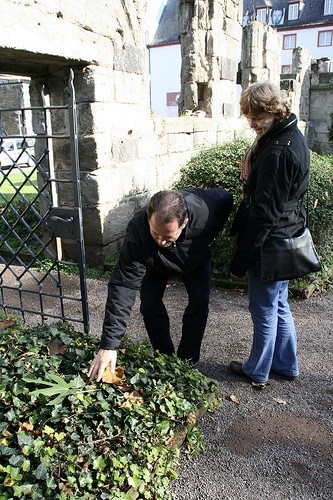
[261,227,322,281]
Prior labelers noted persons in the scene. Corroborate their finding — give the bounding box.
[87,188,232,382]
[229,82,310,383]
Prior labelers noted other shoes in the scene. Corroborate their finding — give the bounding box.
[269,368,298,381]
[230,361,267,386]
